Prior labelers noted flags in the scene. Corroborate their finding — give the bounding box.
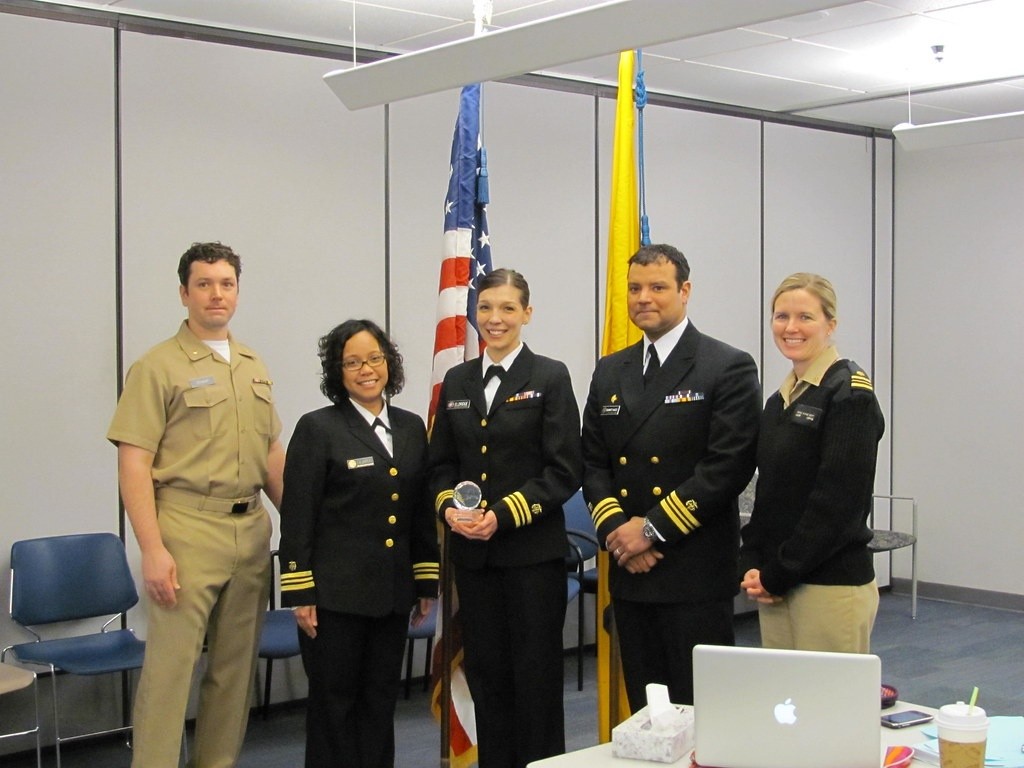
[601,51,641,740]
[431,80,495,768]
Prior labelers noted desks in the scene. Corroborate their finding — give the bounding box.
[524,696,1024,768]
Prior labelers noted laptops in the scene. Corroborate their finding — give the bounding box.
[692,644,881,768]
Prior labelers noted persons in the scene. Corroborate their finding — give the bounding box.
[429,268,582,768]
[581,244,762,715]
[740,274,886,655]
[278,319,439,768]
[107,241,287,768]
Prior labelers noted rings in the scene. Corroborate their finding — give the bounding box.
[617,548,622,555]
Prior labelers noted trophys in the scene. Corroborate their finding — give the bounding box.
[451,480,481,523]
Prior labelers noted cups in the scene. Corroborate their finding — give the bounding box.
[937,704,989,768]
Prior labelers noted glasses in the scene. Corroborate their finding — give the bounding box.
[342,354,386,371]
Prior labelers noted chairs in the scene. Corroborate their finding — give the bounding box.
[0,491,920,768]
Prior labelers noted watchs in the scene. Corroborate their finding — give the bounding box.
[642,517,658,543]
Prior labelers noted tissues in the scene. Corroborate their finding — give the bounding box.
[610,685,694,765]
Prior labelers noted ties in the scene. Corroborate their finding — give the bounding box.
[643,344,660,384]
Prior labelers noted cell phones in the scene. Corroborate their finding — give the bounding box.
[881,710,934,728]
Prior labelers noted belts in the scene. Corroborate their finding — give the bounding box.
[161,490,257,514]
